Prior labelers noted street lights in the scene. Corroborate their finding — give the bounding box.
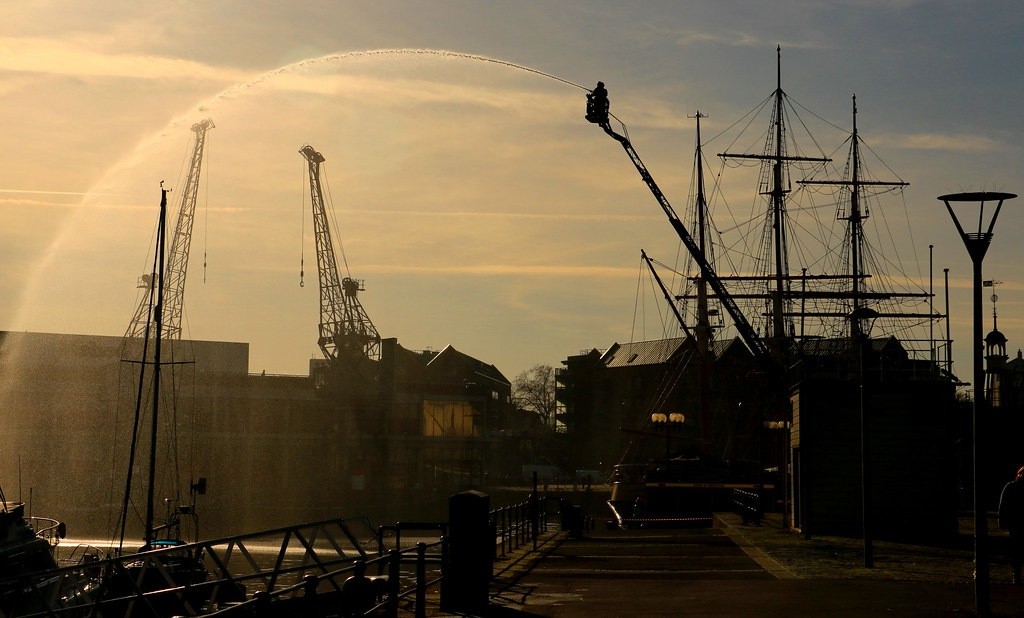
[651,413,685,480]
[769,418,792,472]
[944,191,1017,618]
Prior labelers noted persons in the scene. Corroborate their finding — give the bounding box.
[997,465,1024,583]
[586,473,591,488]
[586,81,608,112]
[581,474,586,489]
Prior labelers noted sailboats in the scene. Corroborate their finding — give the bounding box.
[609,43,979,531]
[34,178,245,618]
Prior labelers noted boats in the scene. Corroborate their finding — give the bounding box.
[0,455,106,618]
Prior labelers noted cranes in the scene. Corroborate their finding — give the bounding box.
[302,145,379,367]
[124,121,207,342]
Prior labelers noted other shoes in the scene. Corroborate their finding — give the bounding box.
[1013,575,1021,585]
